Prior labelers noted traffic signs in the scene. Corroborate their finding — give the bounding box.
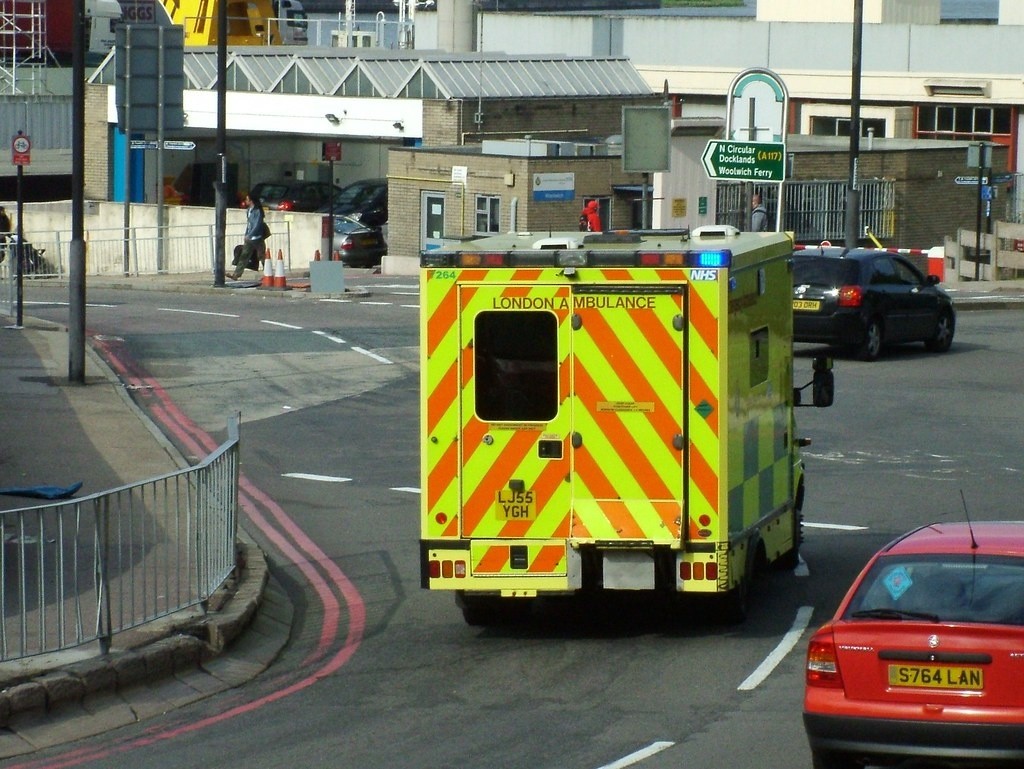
[130,139,196,152]
[954,175,1012,186]
[701,140,787,184]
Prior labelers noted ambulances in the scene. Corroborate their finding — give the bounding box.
[420,225,835,628]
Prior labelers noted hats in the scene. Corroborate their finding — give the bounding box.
[588,201,600,209]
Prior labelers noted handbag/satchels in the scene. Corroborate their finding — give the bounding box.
[231,244,259,271]
[263,222,271,241]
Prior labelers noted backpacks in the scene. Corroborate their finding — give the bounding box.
[578,214,589,231]
[752,209,776,233]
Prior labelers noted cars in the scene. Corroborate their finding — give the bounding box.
[239,178,360,214]
[317,175,389,226]
[802,521,1023,769]
[329,214,389,269]
[790,247,956,360]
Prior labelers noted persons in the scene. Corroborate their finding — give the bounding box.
[226,193,271,280]
[579,200,602,231]
[752,194,768,231]
[0,206,11,263]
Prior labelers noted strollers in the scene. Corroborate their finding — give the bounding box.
[7,232,53,280]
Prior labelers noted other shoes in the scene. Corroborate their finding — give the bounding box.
[225,273,237,281]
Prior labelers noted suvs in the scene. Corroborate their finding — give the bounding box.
[169,160,285,209]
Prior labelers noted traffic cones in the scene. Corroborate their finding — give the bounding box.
[258,248,275,291]
[313,249,321,262]
[271,249,292,292]
